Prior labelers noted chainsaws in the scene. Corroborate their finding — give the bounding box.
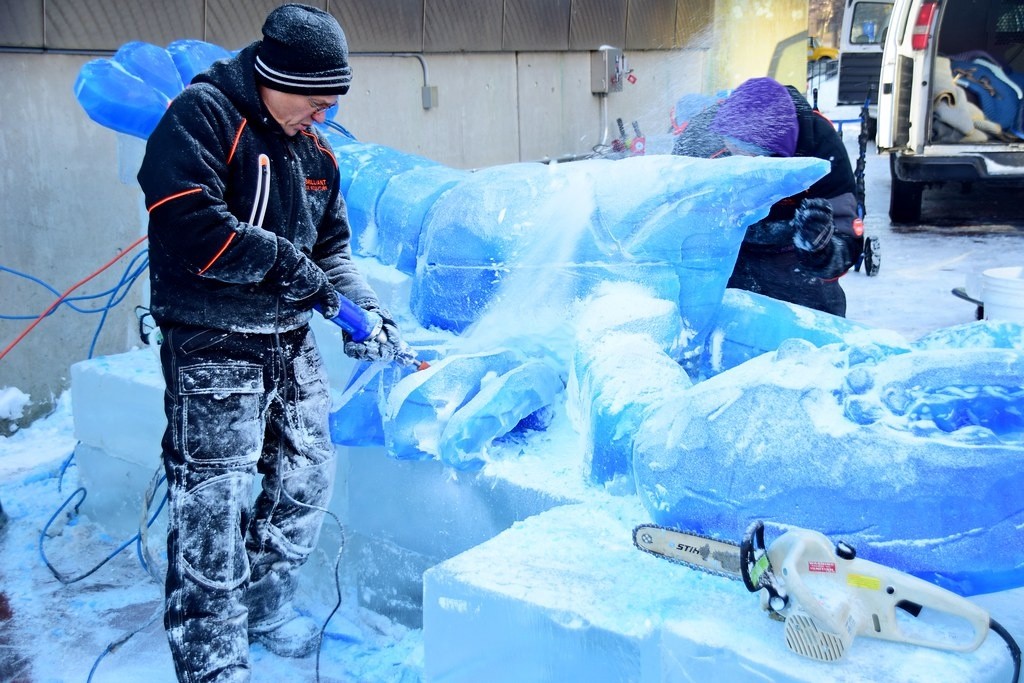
[631,519,989,660]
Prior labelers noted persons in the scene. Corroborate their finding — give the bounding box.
[671,78,864,319]
[136,3,417,683]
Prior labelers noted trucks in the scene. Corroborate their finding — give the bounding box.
[806,35,838,64]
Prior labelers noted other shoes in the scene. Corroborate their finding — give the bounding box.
[248,615,321,658]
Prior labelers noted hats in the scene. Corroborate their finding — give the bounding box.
[723,134,776,157]
[675,94,716,128]
[254,3,353,95]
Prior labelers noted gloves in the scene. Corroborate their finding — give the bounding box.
[282,281,340,319]
[794,198,835,271]
[342,290,418,366]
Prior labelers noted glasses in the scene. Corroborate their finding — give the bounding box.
[306,96,333,113]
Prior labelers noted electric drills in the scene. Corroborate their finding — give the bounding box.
[314,287,430,370]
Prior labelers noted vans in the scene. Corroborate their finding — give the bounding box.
[836,0,1024,226]
